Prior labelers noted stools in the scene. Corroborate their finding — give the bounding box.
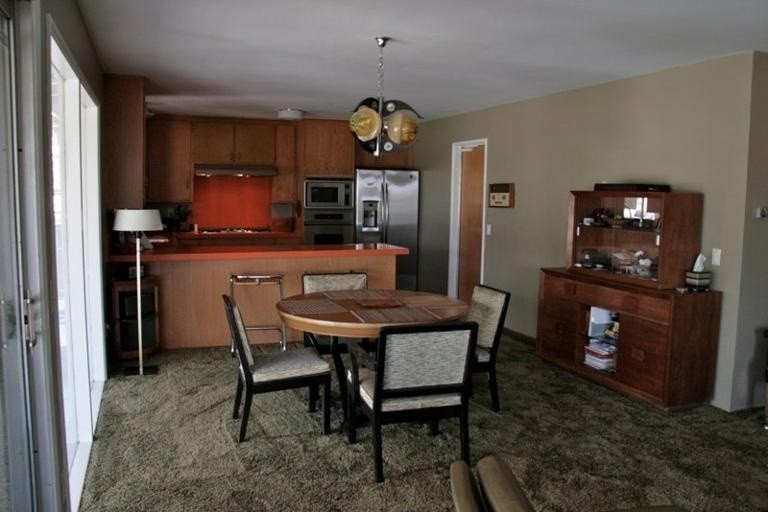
[230,274,287,357]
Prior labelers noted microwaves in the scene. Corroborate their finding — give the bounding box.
[303,180,354,209]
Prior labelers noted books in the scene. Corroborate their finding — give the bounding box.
[582,322,620,373]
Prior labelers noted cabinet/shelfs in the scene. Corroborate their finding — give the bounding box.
[534,268,722,407]
[191,123,276,164]
[273,122,295,202]
[296,119,355,200]
[566,191,704,289]
[113,276,160,359]
[102,77,190,208]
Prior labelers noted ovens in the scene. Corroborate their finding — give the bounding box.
[303,209,354,245]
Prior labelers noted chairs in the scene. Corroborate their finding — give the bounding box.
[348,321,479,484]
[426,282,510,436]
[301,270,369,354]
[222,294,332,443]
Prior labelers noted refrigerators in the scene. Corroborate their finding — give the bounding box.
[355,169,420,292]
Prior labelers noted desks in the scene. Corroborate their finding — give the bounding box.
[276,289,470,423]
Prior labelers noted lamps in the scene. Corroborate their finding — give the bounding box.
[113,208,163,375]
[350,37,424,158]
[278,109,304,122]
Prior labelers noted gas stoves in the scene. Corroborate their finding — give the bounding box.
[199,227,271,234]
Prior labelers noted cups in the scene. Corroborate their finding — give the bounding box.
[583,218,594,225]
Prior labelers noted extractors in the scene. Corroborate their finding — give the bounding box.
[195,164,278,178]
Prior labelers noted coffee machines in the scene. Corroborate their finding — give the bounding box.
[268,200,301,232]
